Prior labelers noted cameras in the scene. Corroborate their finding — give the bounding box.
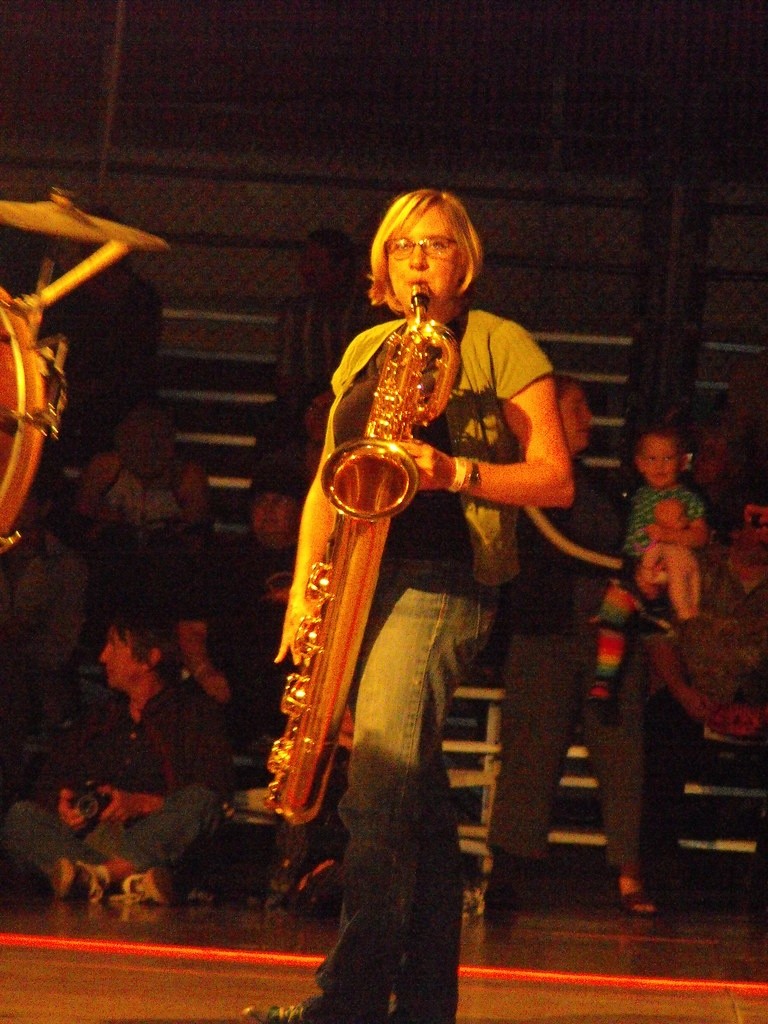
[71,791,112,820]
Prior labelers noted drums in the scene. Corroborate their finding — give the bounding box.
[1,290,71,552]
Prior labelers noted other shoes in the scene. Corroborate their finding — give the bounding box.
[104,874,144,904]
[611,886,659,917]
[51,859,104,906]
[244,1001,313,1024]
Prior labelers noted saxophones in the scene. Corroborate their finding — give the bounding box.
[267,284,463,826]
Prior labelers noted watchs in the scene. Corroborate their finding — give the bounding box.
[466,459,480,495]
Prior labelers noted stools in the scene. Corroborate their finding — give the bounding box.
[439,682,506,927]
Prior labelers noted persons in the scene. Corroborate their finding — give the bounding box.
[242,190,576,1024]
[447,319,768,926]
[0,210,389,921]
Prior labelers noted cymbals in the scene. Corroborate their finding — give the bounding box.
[1,188,169,254]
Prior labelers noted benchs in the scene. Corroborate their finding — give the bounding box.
[4,3,764,854]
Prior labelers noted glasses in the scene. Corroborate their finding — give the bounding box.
[383,237,459,261]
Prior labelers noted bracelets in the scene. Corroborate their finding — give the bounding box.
[449,453,466,496]
